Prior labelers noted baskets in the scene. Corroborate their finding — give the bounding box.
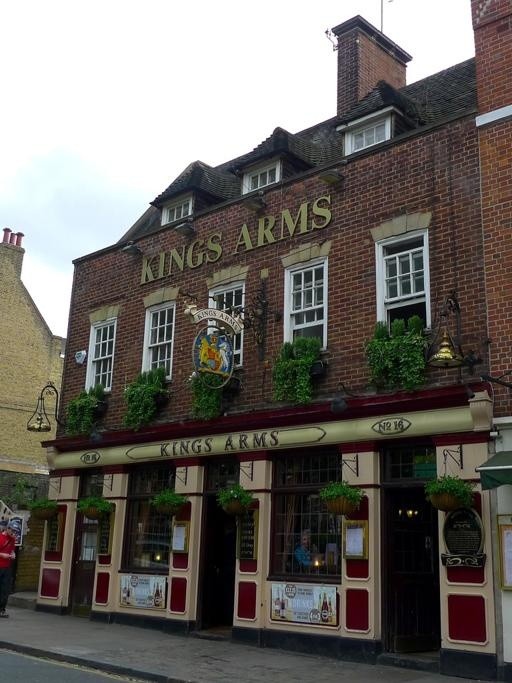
[325,496,356,514]
[222,499,243,512]
[31,507,55,519]
[432,492,464,511]
[83,506,103,519]
[155,504,180,515]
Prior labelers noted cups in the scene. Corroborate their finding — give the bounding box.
[9,550,16,562]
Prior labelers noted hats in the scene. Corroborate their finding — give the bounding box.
[7,521,19,531]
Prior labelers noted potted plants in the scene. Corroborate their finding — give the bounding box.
[320,481,363,515]
[425,471,476,511]
[148,491,187,514]
[218,484,253,515]
[27,498,58,521]
[76,497,111,520]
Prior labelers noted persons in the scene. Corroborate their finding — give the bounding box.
[294,529,325,574]
[0,521,20,618]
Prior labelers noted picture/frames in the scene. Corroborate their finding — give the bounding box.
[343,520,368,561]
[170,521,190,554]
[499,523,512,590]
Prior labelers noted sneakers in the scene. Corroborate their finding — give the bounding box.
[0,611,9,618]
